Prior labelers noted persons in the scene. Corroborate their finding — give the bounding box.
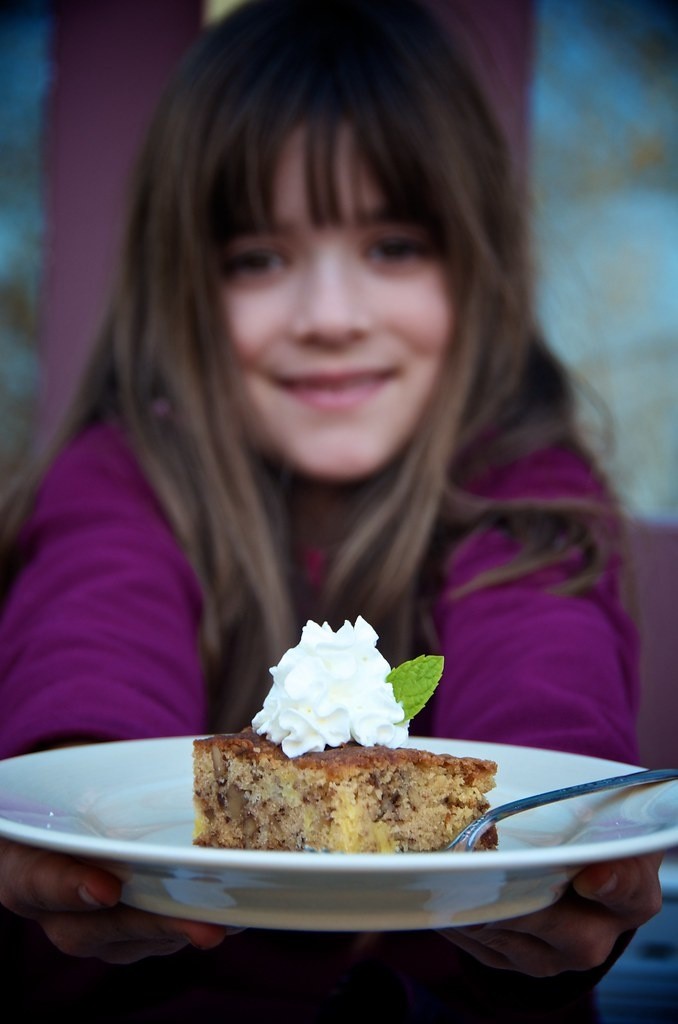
[1,1,665,1024]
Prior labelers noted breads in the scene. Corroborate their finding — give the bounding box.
[192,726,499,856]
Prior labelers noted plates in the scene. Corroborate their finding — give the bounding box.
[0,732,678,932]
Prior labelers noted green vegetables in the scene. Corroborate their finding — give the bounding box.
[386,655,444,726]
